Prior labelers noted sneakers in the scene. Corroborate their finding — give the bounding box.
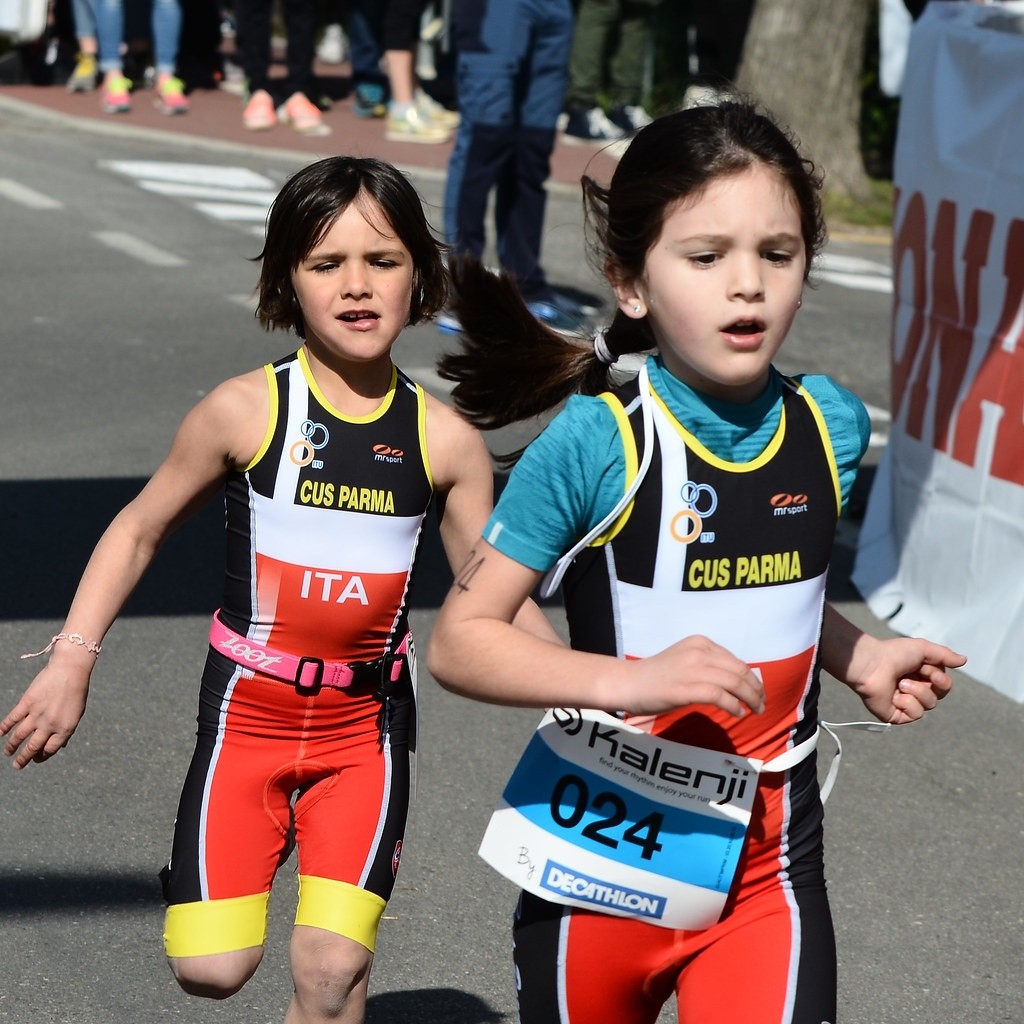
[527,289,590,333]
[279,91,332,137]
[240,89,276,130]
[414,88,460,126]
[561,107,635,150]
[437,306,465,338]
[386,104,447,147]
[610,104,655,134]
[65,52,94,92]
[150,73,188,116]
[355,85,390,116]
[99,73,134,114]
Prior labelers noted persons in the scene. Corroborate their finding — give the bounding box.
[432,1,597,335]
[428,104,966,1024]
[1,0,762,153]
[0,154,563,1022]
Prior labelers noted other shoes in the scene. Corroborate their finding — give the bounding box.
[682,85,737,108]
[319,24,345,64]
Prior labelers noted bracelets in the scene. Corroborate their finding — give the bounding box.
[20,634,102,660]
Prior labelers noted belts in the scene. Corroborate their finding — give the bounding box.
[210,608,412,693]
